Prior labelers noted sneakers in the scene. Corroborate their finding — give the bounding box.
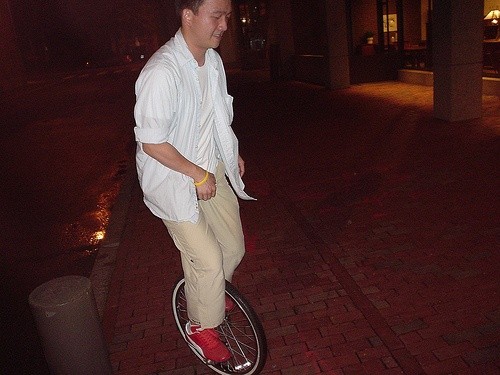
[224,295,235,312]
[185,319,232,363]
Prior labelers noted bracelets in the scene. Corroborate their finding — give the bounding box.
[194,171,209,185]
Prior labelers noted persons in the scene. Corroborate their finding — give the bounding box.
[134,0,258,363]
